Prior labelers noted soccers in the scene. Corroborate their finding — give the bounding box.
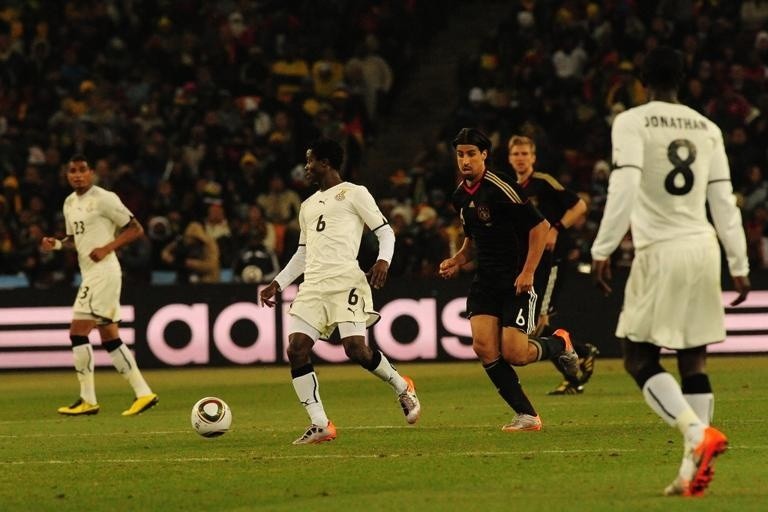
[191,397,231,438]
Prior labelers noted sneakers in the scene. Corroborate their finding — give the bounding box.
[291,419,337,446]
[502,412,544,432]
[58,397,99,414]
[396,375,420,423]
[663,426,728,498]
[551,327,599,394]
[121,393,159,416]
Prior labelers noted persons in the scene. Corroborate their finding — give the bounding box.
[591,54,754,497]
[501,134,601,398]
[438,126,580,434]
[37,153,161,417]
[258,140,421,446]
[1,2,768,292]
